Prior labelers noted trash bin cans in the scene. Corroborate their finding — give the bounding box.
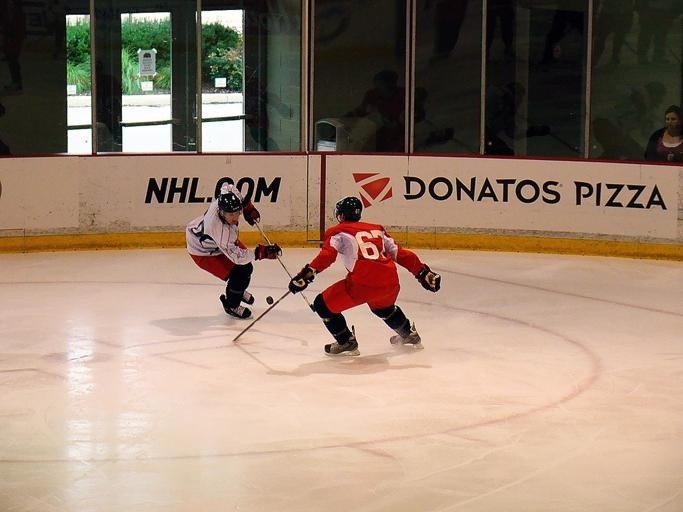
[315,118,350,152]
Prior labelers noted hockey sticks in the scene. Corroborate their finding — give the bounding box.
[254,216,316,312]
[233,290,290,343]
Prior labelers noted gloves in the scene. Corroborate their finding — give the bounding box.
[290,264,317,294]
[257,242,282,260]
[415,264,441,292]
[243,196,261,225]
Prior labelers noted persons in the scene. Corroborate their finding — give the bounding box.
[186,186,280,317]
[286,196,440,355]
[645,106,683,164]
[339,68,405,150]
[478,56,560,156]
[414,87,455,154]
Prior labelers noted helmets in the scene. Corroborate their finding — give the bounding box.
[335,197,363,220]
[217,190,242,213]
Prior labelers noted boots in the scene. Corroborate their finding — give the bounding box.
[242,291,254,304]
[220,294,251,317]
[390,322,421,345]
[324,324,358,353]
